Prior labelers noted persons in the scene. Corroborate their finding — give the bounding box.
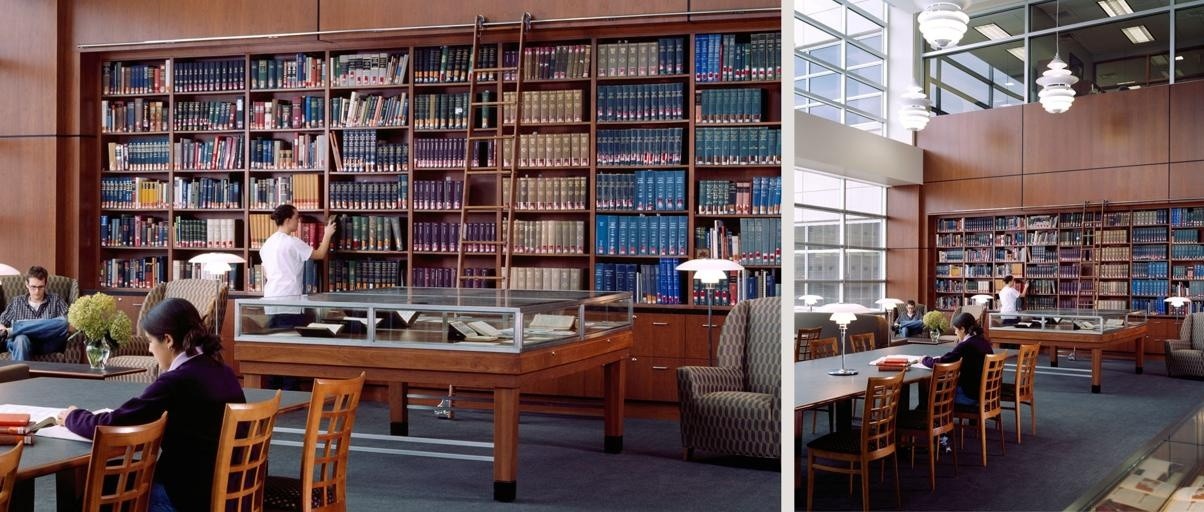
[919,313,995,455]
[259,205,336,391]
[893,301,923,338]
[0,267,70,361]
[56,298,261,512]
[998,277,1029,350]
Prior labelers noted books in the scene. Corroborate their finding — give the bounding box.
[869,354,931,371]
[936,208,1204,316]
[0,404,113,442]
[100,33,781,307]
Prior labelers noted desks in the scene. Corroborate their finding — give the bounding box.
[989,320,1147,393]
[0,376,338,512]
[0,359,147,381]
[230,328,632,501]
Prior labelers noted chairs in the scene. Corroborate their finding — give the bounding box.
[0,439,24,512]
[676,296,780,464]
[82,409,169,512]
[0,275,83,365]
[1164,311,1204,380]
[261,370,366,511]
[888,302,925,338]
[210,389,282,512]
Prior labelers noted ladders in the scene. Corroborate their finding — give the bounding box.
[1077,200,1108,320]
[457,11,534,307]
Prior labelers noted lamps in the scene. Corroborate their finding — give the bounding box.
[897,9,931,132]
[875,298,904,347]
[827,311,858,375]
[917,0,970,50]
[1035,0,1079,116]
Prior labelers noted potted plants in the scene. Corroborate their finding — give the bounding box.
[924,310,949,342]
[67,291,132,370]
